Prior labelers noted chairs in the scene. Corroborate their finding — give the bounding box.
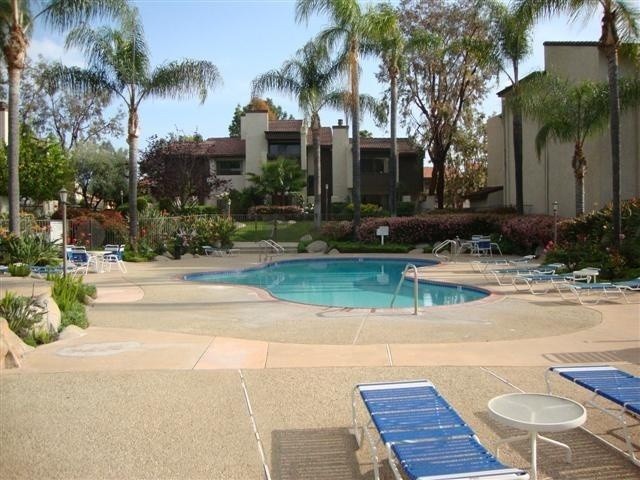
[456,234,502,258]
[65,243,126,275]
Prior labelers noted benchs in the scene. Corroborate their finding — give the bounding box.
[469,254,640,306]
[352,378,529,480]
[545,363,640,469]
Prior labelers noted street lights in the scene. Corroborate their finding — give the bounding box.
[58,186,68,280]
[552,200,559,241]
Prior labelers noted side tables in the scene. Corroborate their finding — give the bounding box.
[487,393,588,465]
